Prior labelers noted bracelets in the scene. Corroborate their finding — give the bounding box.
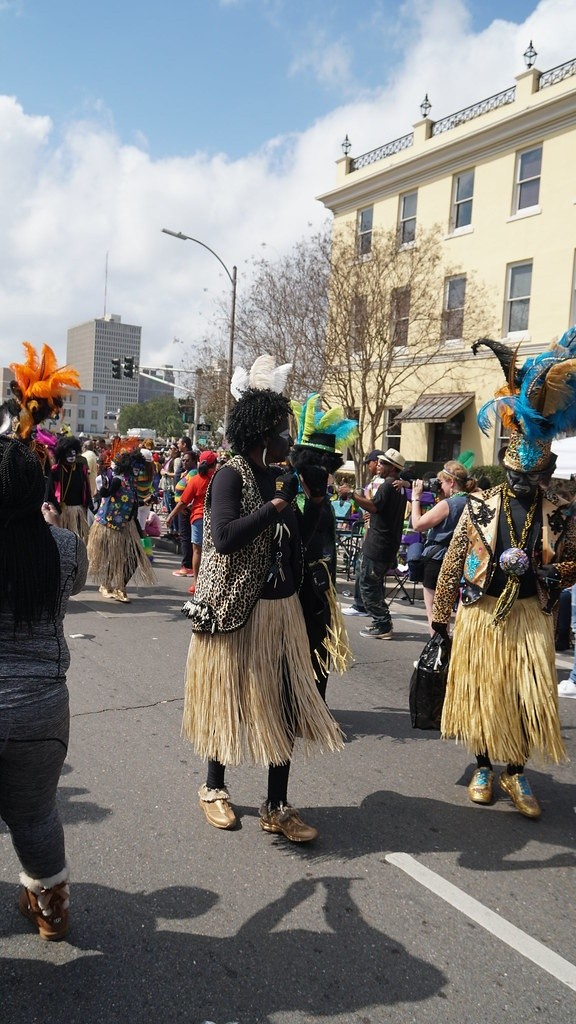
[412,499,420,502]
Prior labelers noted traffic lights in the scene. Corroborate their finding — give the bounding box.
[111,358,122,379]
[178,398,195,423]
[123,356,135,379]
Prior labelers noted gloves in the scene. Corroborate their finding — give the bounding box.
[275,474,298,503]
[432,621,447,639]
[537,564,561,590]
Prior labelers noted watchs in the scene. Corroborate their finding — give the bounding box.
[347,490,354,499]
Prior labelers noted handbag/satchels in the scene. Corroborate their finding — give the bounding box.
[408,543,424,581]
[409,629,452,730]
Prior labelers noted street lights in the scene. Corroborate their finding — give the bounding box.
[161,226,238,443]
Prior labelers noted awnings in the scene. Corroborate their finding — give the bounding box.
[392,392,474,424]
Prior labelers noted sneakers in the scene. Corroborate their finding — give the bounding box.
[115,589,130,603]
[259,800,318,842]
[497,770,541,818]
[99,584,114,598]
[468,766,494,803]
[198,783,236,829]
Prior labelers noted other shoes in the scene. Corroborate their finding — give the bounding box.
[341,607,369,617]
[173,567,194,577]
[359,623,393,639]
[557,680,576,699]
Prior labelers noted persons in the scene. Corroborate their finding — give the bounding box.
[0,442,90,941]
[431,434,576,818]
[187,389,320,842]
[42,437,576,699]
[296,461,331,728]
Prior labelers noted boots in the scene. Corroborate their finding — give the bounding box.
[17,868,70,941]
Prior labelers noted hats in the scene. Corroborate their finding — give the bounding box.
[199,451,218,465]
[471,325,576,473]
[290,394,359,458]
[361,450,384,462]
[378,447,405,471]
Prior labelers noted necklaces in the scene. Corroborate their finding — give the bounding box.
[491,483,538,625]
